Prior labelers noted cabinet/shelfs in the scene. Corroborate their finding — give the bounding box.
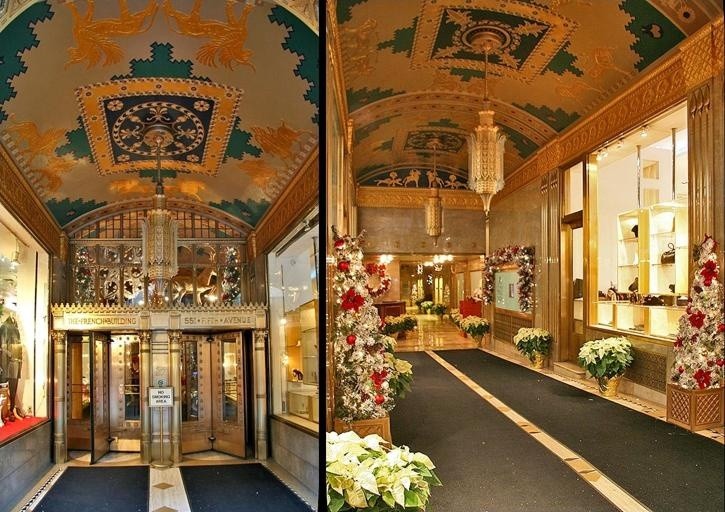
[615,208,649,335]
[649,204,690,340]
[283,298,319,424]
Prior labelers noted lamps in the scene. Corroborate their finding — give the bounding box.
[104,331,114,343]
[594,124,648,162]
[469,30,505,221]
[139,136,178,303]
[205,329,214,342]
[9,238,21,272]
[423,138,445,247]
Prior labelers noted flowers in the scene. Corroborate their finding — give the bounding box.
[576,336,635,379]
[325,225,532,512]
[514,326,553,360]
[669,232,725,391]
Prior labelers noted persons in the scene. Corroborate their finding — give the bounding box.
[0,312,24,420]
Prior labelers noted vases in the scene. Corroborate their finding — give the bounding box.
[529,350,545,369]
[666,382,725,432]
[594,375,623,397]
